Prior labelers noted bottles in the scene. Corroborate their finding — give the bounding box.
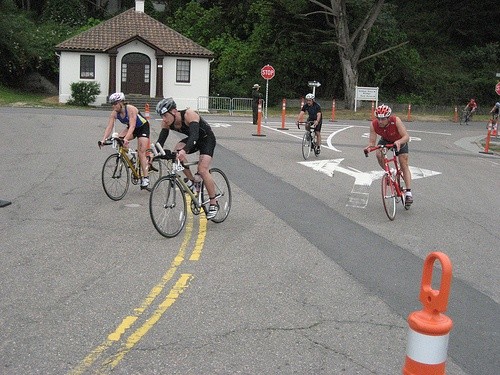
[194,171,201,192]
[184,178,196,193]
[126,152,137,166]
[390,168,395,176]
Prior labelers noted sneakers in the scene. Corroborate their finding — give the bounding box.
[206,200,220,220]
[182,179,195,191]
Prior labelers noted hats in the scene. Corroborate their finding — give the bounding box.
[252,84,261,88]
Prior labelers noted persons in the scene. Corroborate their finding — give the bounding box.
[462,99,500,128]
[144,97,220,219]
[296,94,322,154]
[361,105,413,205]
[252,84,262,125]
[98,93,151,187]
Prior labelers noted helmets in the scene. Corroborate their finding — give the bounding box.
[306,93,315,100]
[109,92,125,103]
[376,105,392,117]
[156,97,174,114]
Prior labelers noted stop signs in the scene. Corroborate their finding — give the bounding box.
[261,65,276,80]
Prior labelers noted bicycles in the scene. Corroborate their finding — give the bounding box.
[296,121,321,161]
[362,143,411,221]
[486,111,500,129]
[142,148,232,238]
[97,134,163,201]
[462,110,474,126]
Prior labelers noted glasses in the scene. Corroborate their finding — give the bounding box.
[110,102,117,106]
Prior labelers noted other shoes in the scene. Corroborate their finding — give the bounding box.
[142,177,149,186]
[316,145,320,154]
[405,194,413,204]
